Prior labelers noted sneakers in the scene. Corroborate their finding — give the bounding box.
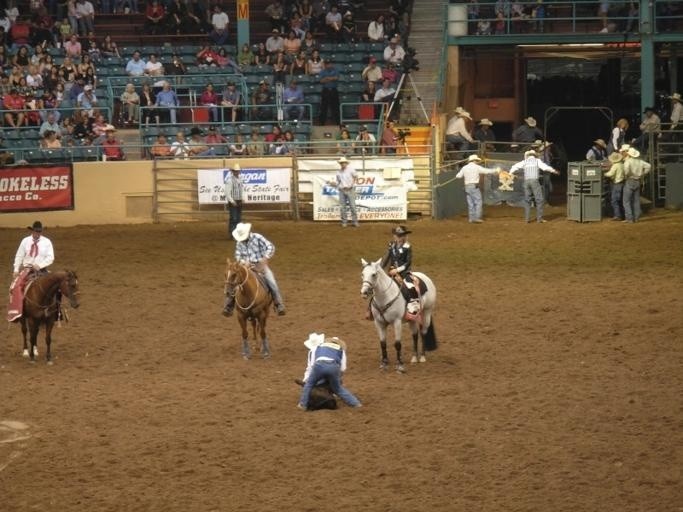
[473,219,484,222]
[408,303,418,314]
[276,303,286,315]
[610,216,623,221]
[354,403,362,408]
[621,220,634,224]
[223,305,234,318]
[298,404,308,410]
[537,219,547,223]
[634,219,639,223]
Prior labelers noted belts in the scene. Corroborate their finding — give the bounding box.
[318,360,338,365]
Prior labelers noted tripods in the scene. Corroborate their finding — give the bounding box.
[397,138,410,156]
[384,71,431,128]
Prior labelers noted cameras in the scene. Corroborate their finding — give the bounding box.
[398,128,411,138]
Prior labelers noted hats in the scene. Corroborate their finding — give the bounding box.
[643,107,655,113]
[452,107,465,113]
[671,93,683,102]
[524,117,537,127]
[28,222,47,232]
[337,157,351,165]
[326,336,347,350]
[625,148,640,158]
[230,164,241,171]
[210,124,215,131]
[458,112,472,120]
[85,85,92,92]
[227,81,235,86]
[324,58,331,63]
[393,226,411,235]
[190,128,203,134]
[593,139,607,149]
[619,144,630,151]
[608,152,623,162]
[304,333,325,349]
[524,150,538,159]
[370,57,376,64]
[232,223,252,242]
[479,118,493,126]
[466,155,481,163]
[390,38,397,44]
[272,29,279,33]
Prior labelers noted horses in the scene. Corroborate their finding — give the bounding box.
[360,257,437,375]
[223,257,274,360]
[20,268,81,367]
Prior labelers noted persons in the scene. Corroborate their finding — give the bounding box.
[222,222,286,317]
[446,93,682,224]
[225,163,244,239]
[2,0,408,161]
[297,336,363,411]
[10,221,55,323]
[365,226,421,321]
[329,157,361,228]
[469,1,682,37]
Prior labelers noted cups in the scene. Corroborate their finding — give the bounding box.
[102,155,106,161]
